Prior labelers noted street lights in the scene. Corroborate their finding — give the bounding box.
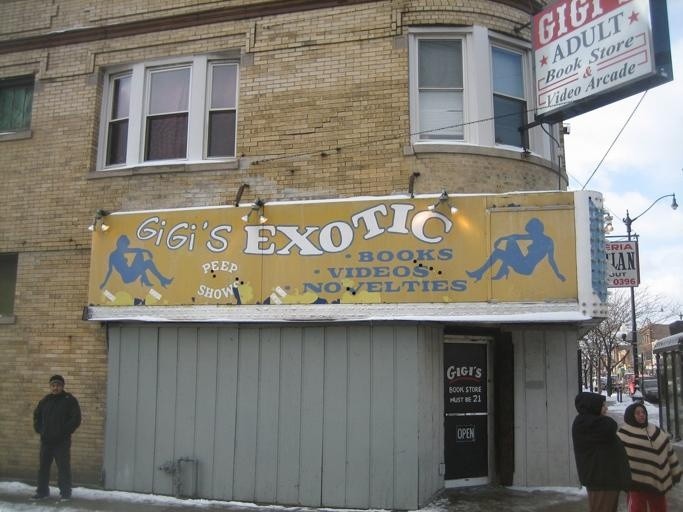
[621,193,678,405]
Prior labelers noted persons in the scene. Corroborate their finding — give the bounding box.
[28,373,80,503]
[463,216,566,285]
[613,403,682,511]
[571,391,633,512]
[97,233,174,290]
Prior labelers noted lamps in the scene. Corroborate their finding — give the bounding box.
[427,193,458,216]
[241,199,269,225]
[602,210,615,233]
[88,209,110,232]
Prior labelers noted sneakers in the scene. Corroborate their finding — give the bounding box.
[28,493,50,501]
[55,494,73,504]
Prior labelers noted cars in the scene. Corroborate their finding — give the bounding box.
[589,370,678,406]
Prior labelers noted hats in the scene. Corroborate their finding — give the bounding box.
[49,375,64,385]
